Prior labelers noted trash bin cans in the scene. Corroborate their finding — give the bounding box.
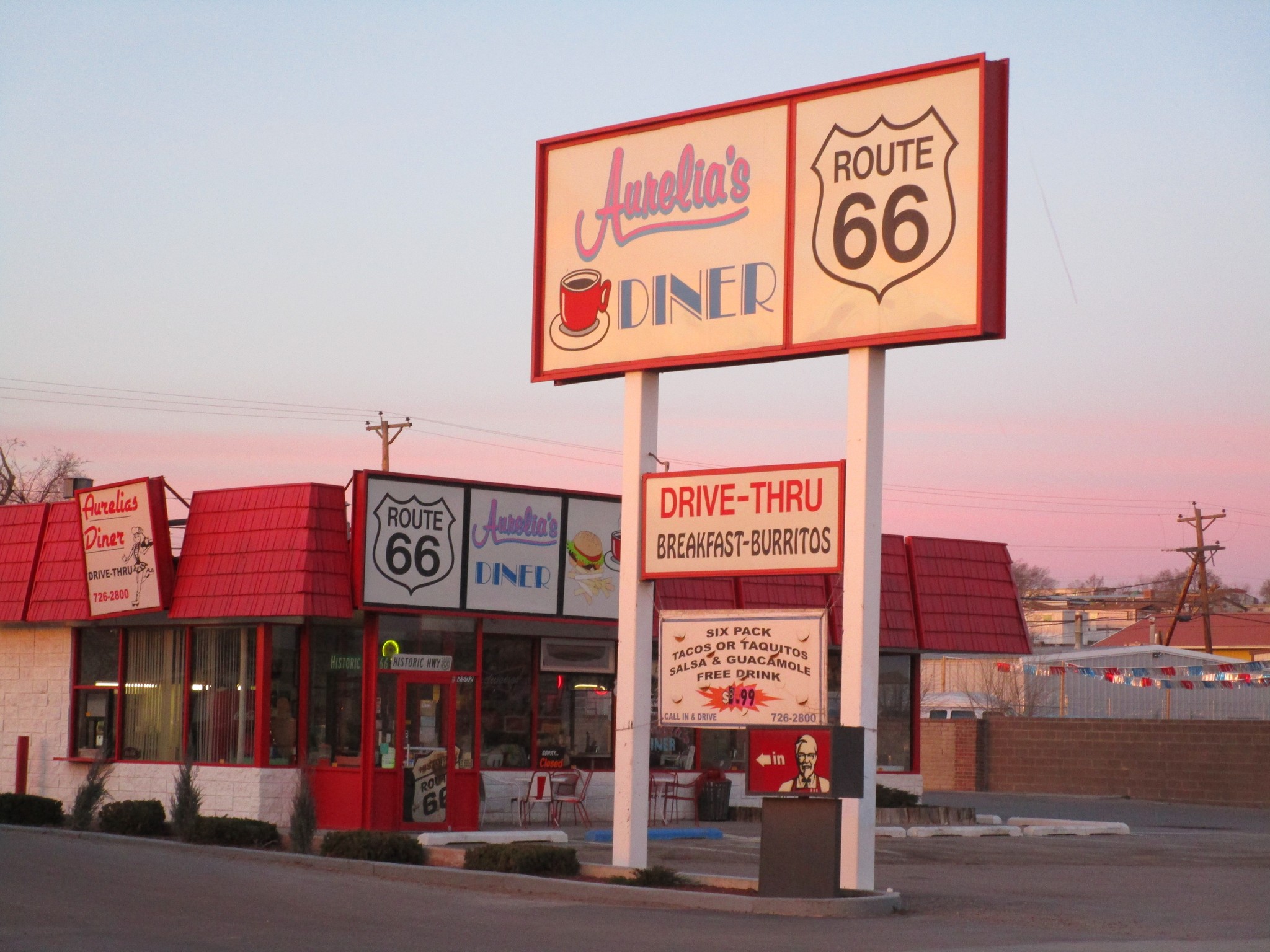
[697,780,733,821]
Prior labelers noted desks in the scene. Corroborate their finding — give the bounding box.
[515,774,569,827]
[651,777,681,826]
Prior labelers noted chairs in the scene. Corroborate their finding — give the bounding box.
[480,770,520,829]
[662,769,713,826]
[520,769,558,828]
[553,769,595,827]
[547,768,583,826]
[650,768,680,824]
